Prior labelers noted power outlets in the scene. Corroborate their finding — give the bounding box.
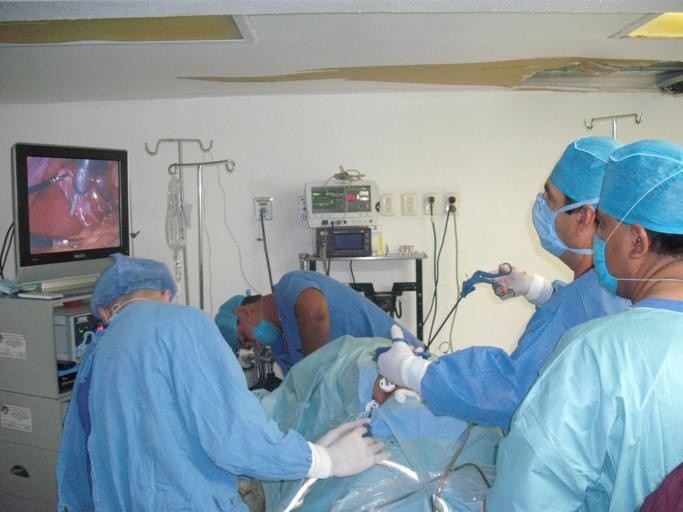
[254,197,273,221]
[443,192,460,216]
[400,193,417,216]
[422,192,438,215]
[379,194,396,217]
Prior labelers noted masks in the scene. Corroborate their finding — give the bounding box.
[591,218,649,298]
[530,193,595,258]
[235,307,282,346]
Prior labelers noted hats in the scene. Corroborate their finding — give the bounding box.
[550,135,624,211]
[213,295,245,350]
[597,139,683,235]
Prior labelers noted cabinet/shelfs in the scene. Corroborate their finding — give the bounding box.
[0,286,110,511]
[298,250,428,343]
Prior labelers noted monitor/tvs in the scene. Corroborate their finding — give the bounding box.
[10,142,130,293]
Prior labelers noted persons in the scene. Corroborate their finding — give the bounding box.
[55,249,394,512]
[483,138,682,512]
[376,134,633,427]
[213,268,431,377]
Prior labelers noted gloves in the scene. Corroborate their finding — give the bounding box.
[483,263,554,307]
[375,324,431,393]
[306,418,397,478]
